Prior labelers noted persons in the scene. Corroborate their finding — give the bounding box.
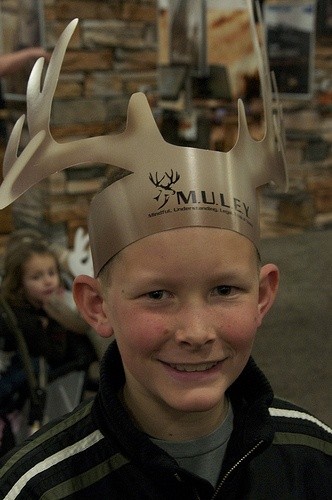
[1,226,95,453]
[1,138,332,500]
